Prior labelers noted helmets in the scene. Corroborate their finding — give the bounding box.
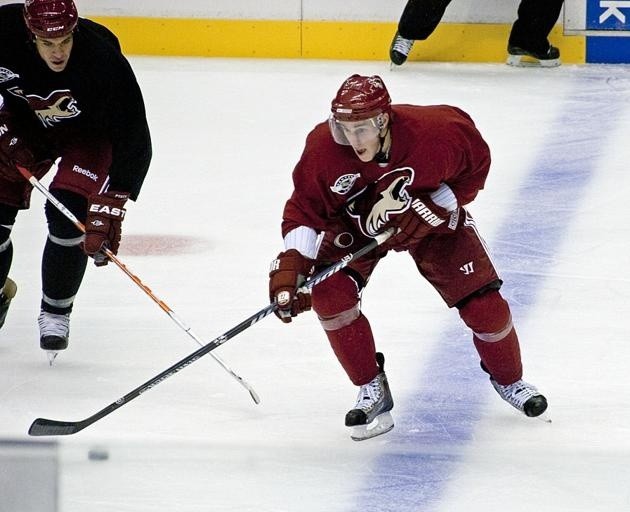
[328,74,392,145]
[22,0,78,36]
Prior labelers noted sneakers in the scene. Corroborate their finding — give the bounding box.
[345,352,394,427]
[479,359,548,417]
[39,294,75,350]
[390,30,414,65]
[507,37,560,60]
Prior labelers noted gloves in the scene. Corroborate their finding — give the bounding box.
[269,250,311,322]
[376,194,453,253]
[0,121,36,173]
[79,191,130,267]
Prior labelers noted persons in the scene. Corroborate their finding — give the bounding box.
[389,1,564,70]
[0,1,152,369]
[270,74,551,440]
[0,0,25,44]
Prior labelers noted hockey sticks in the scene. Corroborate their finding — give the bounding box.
[27,228,404,435]
[17,163,260,405]
